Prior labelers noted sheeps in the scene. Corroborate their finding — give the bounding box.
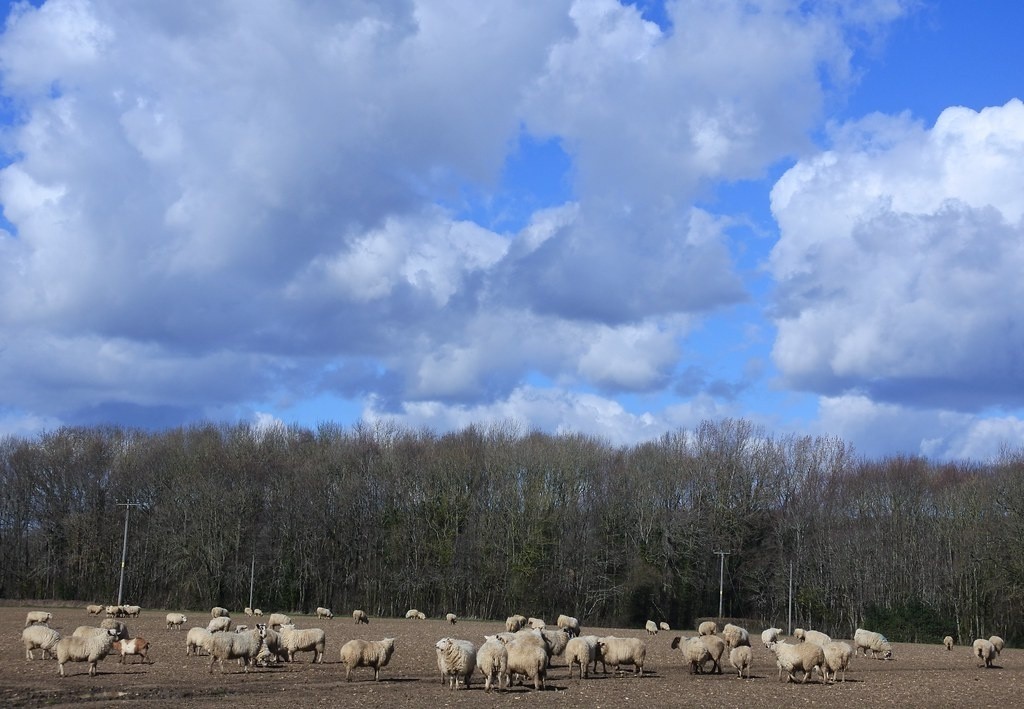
[853,627,893,661]
[405,609,426,620]
[187,607,325,674]
[339,637,395,682]
[446,614,457,625]
[165,612,187,630]
[18,604,153,678]
[353,610,369,625]
[660,621,670,631]
[973,635,1004,668]
[761,627,852,685]
[476,614,647,692]
[943,635,953,651]
[671,621,753,680]
[317,607,334,620]
[646,619,658,636]
[434,637,476,690]
